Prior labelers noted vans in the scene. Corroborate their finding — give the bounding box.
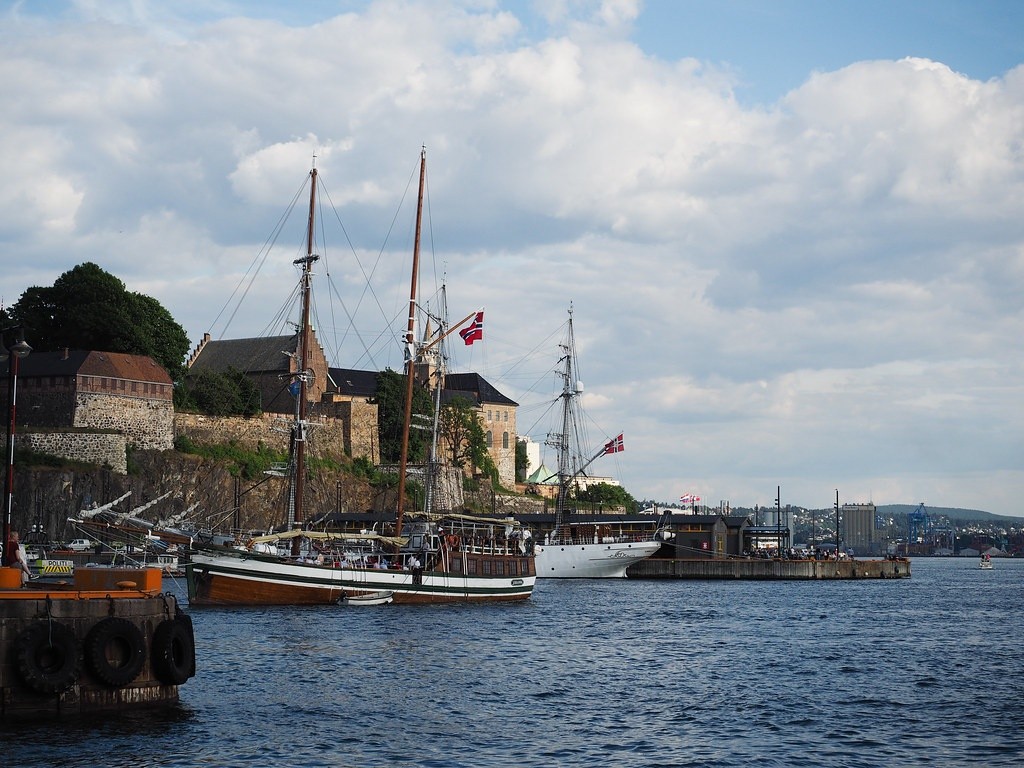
[64,539,90,552]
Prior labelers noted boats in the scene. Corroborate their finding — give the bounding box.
[978,554,993,569]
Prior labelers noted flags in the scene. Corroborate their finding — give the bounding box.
[680,493,700,504]
[601,435,624,457]
[459,307,485,344]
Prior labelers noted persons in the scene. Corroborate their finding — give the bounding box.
[2,530,39,587]
[374,557,387,569]
[523,526,531,540]
[422,538,430,549]
[297,554,324,566]
[509,527,520,537]
[408,556,421,569]
[743,545,854,561]
[981,553,990,562]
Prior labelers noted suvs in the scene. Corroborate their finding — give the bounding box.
[50,543,57,552]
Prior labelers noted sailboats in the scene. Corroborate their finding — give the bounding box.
[430,298,660,579]
[65,146,540,607]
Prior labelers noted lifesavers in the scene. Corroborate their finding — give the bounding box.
[6,617,83,700]
[153,614,196,685]
[449,535,459,548]
[84,616,147,685]
